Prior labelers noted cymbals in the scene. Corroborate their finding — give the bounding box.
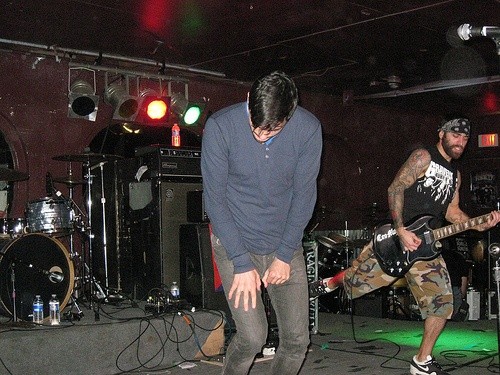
[52,177,86,187]
[53,153,126,162]
[0,166,30,182]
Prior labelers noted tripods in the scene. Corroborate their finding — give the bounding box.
[66,160,139,321]
[385,287,421,322]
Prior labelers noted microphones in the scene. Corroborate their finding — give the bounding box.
[457,23,500,41]
[45,172,53,196]
[51,274,65,283]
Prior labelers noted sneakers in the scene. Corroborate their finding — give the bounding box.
[410,355,451,375]
[308,277,338,301]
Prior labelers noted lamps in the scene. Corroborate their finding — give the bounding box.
[64,67,99,121]
[166,78,202,126]
[101,70,144,122]
[135,76,172,124]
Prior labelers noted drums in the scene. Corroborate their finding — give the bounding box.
[26,197,76,238]
[317,231,368,270]
[0,217,27,240]
[0,231,75,324]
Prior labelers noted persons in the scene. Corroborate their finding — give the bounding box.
[307,112,500,375]
[200,73,323,375]
[441,250,468,319]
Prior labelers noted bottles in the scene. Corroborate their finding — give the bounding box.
[49,294,61,326]
[169,282,181,311]
[32,295,43,327]
[171,123,180,146]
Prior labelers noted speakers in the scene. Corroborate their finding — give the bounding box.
[129,181,280,331]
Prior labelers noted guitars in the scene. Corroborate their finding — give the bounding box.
[371,213,493,280]
